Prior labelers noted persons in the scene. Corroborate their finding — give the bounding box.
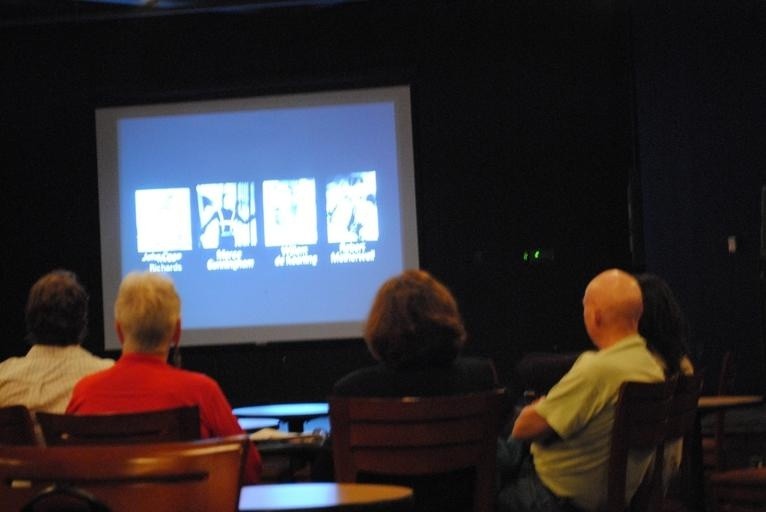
[485,269,667,511]
[59,270,264,486]
[320,269,497,512]
[0,270,116,490]
[631,273,698,512]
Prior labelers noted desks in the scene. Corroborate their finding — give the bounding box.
[240,480,413,512]
[696,392,762,445]
[327,384,521,489]
[231,401,329,434]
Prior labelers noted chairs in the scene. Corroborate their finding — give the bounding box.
[658,370,702,503]
[0,403,36,443]
[604,363,674,509]
[2,438,248,510]
[31,409,203,445]
